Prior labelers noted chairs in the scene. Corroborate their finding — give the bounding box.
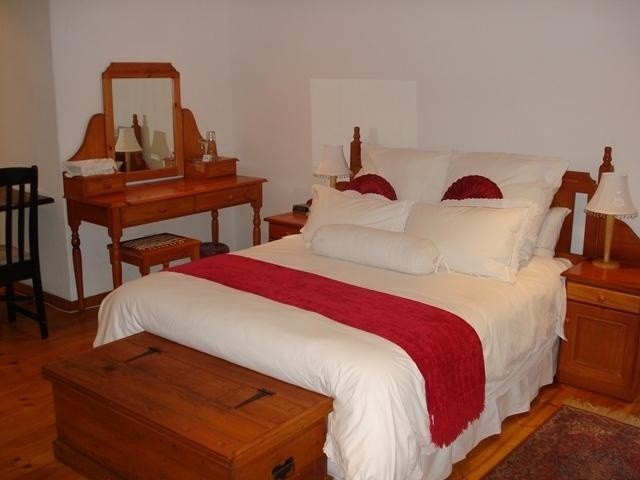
[0,165,49,338]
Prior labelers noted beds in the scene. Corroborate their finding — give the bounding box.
[116,114,150,172]
[314,144,354,187]
[93,125,640,480]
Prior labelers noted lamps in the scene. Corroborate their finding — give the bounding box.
[115,128,143,171]
[584,172,638,270]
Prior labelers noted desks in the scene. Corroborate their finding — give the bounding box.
[63,175,268,310]
[0,187,55,214]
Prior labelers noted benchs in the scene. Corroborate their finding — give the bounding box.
[42,332,334,479]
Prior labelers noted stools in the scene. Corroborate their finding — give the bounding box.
[106,232,202,290]
[201,242,229,257]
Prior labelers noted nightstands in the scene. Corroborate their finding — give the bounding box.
[557,258,640,403]
[264,210,309,242]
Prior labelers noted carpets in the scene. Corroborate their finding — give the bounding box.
[481,405,640,480]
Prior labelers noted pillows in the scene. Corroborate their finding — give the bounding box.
[311,224,442,275]
[406,202,530,284]
[302,184,413,249]
[534,206,572,258]
[441,148,569,265]
[355,141,453,204]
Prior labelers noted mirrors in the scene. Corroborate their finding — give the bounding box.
[102,61,185,183]
